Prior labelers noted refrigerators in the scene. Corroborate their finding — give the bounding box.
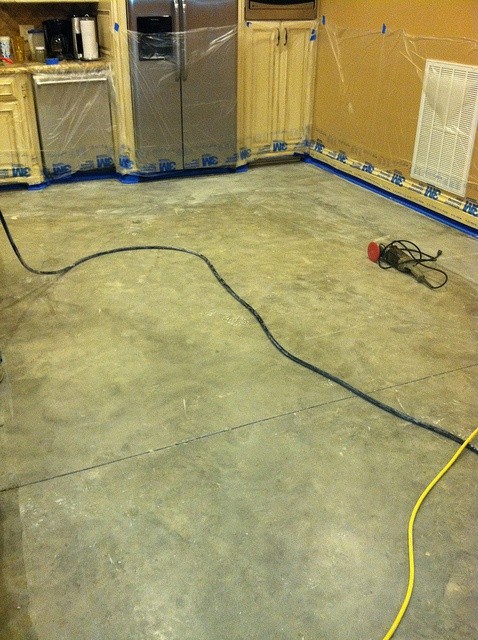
[126,0,240,171]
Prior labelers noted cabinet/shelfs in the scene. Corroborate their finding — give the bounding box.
[0,78,47,190]
[248,21,316,166]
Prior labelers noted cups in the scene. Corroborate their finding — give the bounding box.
[8,36,27,63]
[0,36,12,59]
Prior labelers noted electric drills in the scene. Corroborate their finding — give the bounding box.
[367,239,448,289]
